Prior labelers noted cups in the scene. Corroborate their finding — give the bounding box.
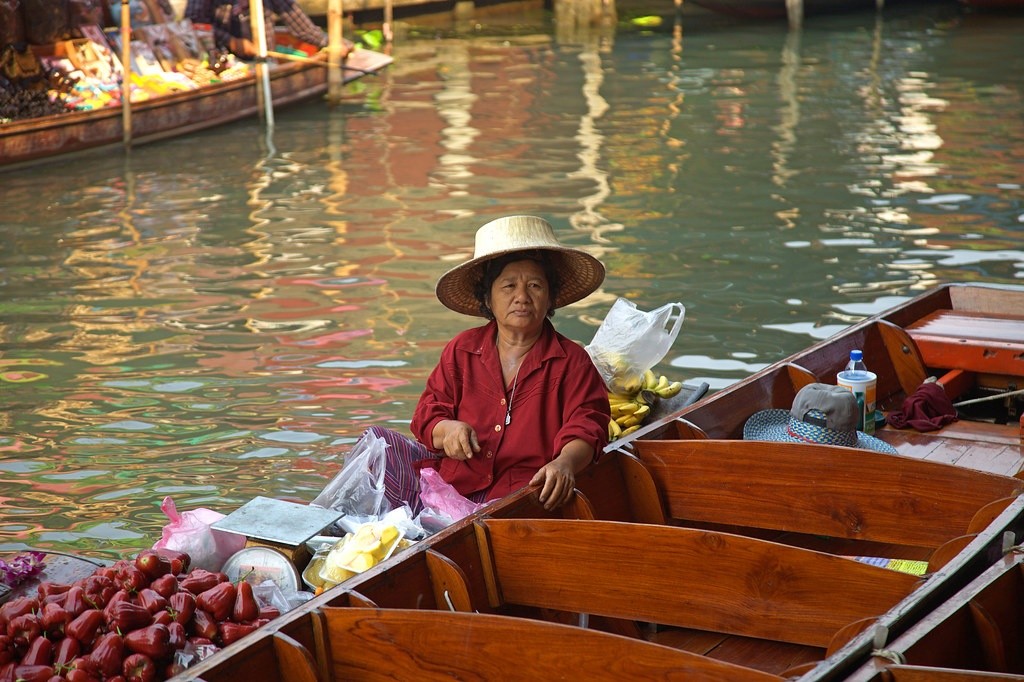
[836,371,877,437]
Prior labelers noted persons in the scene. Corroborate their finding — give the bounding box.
[408,215,612,514]
[183,0,355,62]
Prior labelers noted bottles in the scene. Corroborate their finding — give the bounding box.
[845,350,868,381]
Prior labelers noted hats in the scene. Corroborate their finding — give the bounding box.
[743,382,900,457]
[436,215,606,318]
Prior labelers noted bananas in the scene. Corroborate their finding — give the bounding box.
[595,350,681,441]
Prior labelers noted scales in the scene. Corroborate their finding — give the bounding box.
[210,495,346,596]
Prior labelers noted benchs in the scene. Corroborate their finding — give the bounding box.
[905,307,1024,379]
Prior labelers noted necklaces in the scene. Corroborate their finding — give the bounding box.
[496,335,525,425]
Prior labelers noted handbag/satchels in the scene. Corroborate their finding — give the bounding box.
[584,297,685,398]
[149,496,248,574]
[310,430,394,538]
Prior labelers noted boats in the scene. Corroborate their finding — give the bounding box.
[162,283,1024,682]
[0,25,394,170]
